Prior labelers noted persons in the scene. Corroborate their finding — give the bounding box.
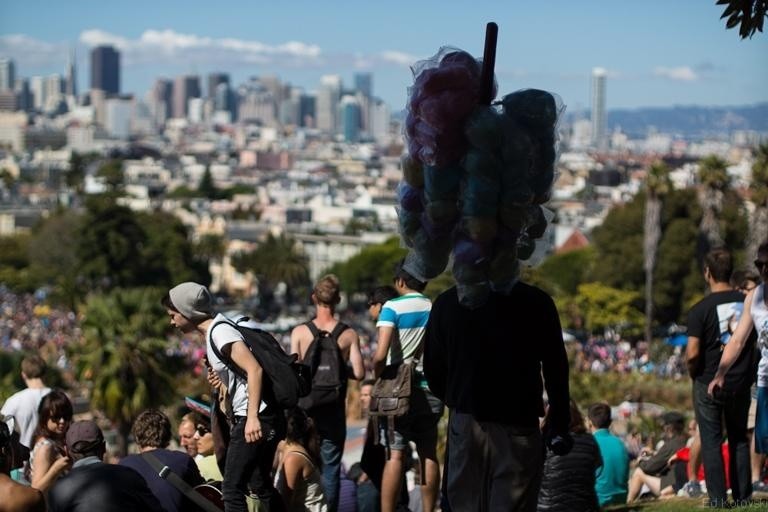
[0,248,768,512]
[422,220,573,510]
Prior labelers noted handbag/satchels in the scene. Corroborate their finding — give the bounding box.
[193,479,224,512]
[367,363,418,417]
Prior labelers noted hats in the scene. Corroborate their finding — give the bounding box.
[65,420,104,453]
[169,281,213,320]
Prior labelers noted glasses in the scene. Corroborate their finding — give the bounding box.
[195,426,209,437]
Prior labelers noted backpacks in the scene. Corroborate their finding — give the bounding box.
[209,316,300,408]
[302,321,349,406]
